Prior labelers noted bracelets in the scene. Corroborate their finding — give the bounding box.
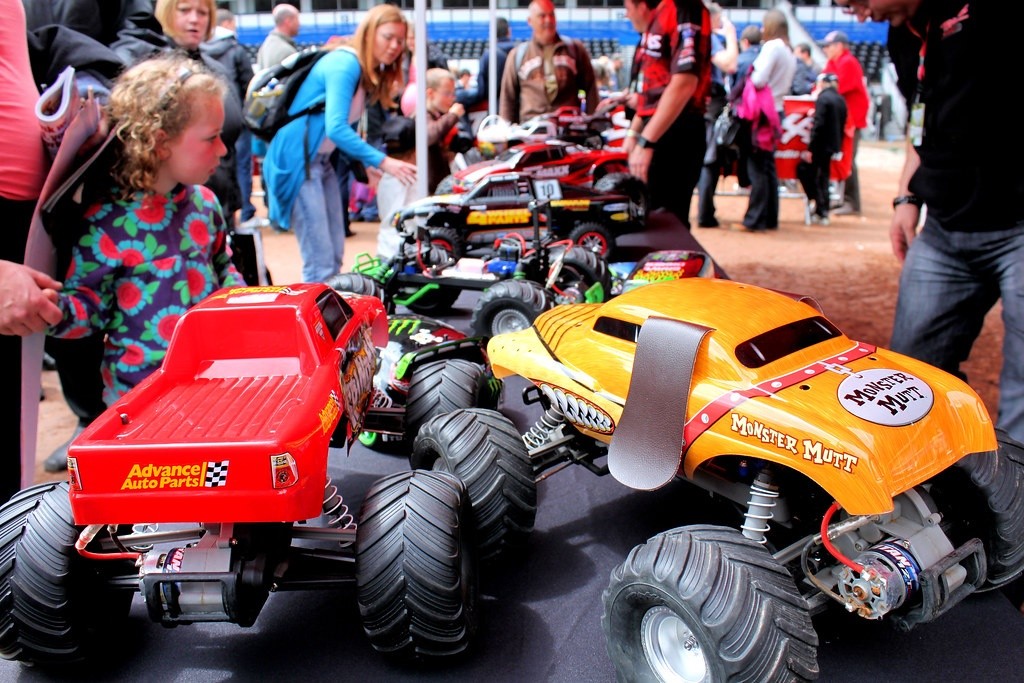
[625,130,640,138]
[892,196,924,209]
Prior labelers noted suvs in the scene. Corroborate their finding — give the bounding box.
[405,268,1023,683]
[330,76,737,367]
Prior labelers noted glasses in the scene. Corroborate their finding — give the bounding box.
[841,0,860,15]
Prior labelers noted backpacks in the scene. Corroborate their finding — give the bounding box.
[242,44,362,140]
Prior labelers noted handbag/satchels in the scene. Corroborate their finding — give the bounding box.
[712,103,746,151]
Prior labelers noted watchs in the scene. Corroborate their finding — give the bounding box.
[637,136,656,148]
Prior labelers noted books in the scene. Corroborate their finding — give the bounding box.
[36,64,81,156]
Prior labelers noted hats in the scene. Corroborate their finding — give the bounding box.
[816,31,849,46]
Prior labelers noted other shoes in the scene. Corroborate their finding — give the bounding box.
[835,202,858,215]
[42,425,84,474]
[731,223,757,233]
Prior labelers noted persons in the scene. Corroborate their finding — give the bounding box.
[835,0,1023,449]
[592,0,712,228]
[0,0,62,505]
[696,0,869,232]
[25,0,522,473]
[499,0,600,126]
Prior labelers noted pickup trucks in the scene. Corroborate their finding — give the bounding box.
[0,280,506,671]
